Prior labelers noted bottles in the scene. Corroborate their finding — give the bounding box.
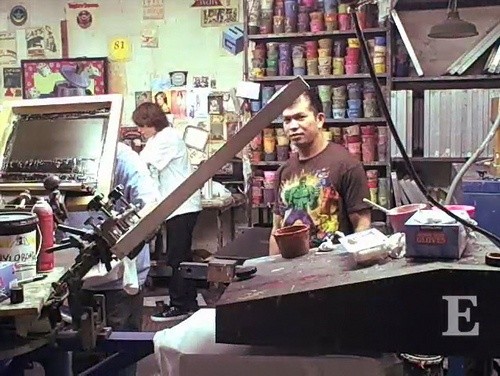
[31,197,55,273]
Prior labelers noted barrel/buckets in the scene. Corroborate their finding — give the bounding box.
[0,211,43,280]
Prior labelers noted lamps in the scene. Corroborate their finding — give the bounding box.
[428,0,479,39]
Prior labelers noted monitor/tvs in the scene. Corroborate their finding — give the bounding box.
[0,106,110,184]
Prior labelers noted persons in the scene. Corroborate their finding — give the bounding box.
[268,90,373,258]
[133,104,204,322]
[111,141,159,281]
[135,90,187,117]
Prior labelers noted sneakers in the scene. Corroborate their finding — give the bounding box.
[150,305,188,322]
[187,305,199,316]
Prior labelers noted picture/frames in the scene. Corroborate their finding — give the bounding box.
[21,57,109,99]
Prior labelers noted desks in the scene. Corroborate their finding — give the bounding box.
[153,309,403,376]
[0,266,65,316]
[200,193,244,251]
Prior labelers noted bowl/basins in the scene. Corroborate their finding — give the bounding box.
[248,0,379,34]
[272,224,310,258]
[252,36,386,77]
[250,124,387,161]
[250,83,386,119]
[387,202,427,233]
[250,168,388,209]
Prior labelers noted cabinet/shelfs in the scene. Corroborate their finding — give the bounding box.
[243,0,500,244]
